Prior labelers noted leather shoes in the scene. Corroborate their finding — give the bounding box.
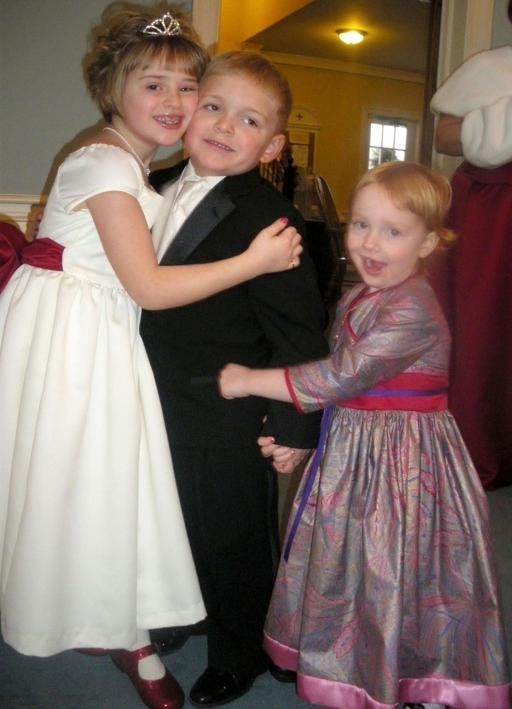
[191,659,269,707]
[111,646,186,709]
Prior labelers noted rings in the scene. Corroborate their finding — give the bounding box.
[289,258,293,267]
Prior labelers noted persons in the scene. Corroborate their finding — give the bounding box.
[1,1,302,707]
[218,157,511,709]
[23,47,334,707]
[430,1,511,490]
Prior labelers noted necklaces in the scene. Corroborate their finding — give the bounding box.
[100,126,152,176]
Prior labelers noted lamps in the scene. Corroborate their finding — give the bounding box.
[334,28,368,45]
